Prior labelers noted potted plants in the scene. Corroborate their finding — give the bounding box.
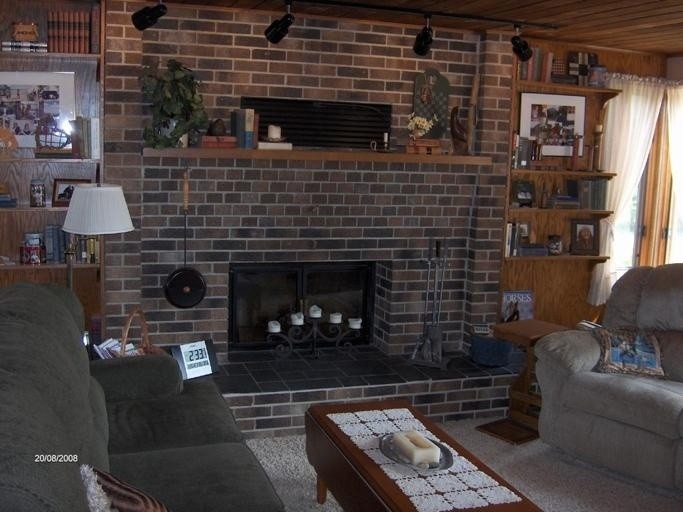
[130,55,207,151]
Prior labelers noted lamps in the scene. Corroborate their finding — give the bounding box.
[125,0,538,65]
[54,181,138,343]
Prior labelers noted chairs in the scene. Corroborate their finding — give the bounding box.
[529,259,682,498]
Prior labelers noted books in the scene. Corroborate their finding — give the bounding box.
[200,108,260,150]
[92,337,141,359]
[566,176,607,212]
[43,224,102,265]
[1,0,101,54]
[515,41,554,86]
[71,116,101,160]
[505,219,519,259]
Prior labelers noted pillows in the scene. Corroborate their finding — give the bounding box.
[586,319,667,381]
[74,456,171,512]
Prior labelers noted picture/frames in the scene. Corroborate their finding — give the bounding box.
[49,177,93,207]
[515,89,586,160]
[566,217,602,257]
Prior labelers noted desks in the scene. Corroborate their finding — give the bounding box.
[485,318,568,435]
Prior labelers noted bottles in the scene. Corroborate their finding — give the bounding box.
[589,64,607,89]
[29,178,45,208]
[547,234,564,255]
[18,240,46,265]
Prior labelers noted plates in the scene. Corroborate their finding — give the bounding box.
[261,136,288,143]
[378,432,454,472]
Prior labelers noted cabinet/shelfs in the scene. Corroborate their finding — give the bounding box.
[0,44,105,271]
[500,77,622,264]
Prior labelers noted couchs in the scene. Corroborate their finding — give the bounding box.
[0,274,293,512]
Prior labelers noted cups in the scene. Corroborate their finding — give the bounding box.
[347,318,362,329]
[268,126,280,139]
[267,320,281,332]
[329,313,342,323]
[290,312,304,326]
[307,305,321,317]
